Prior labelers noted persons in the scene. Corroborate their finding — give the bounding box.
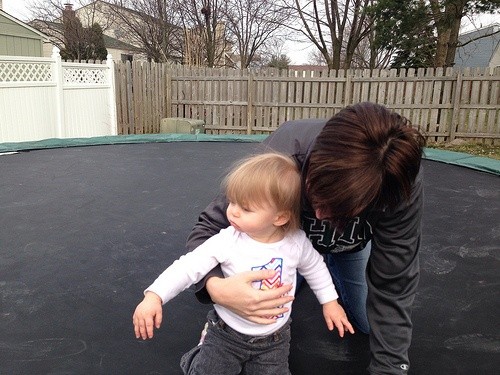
[131,146,356,375]
[184,99,428,375]
